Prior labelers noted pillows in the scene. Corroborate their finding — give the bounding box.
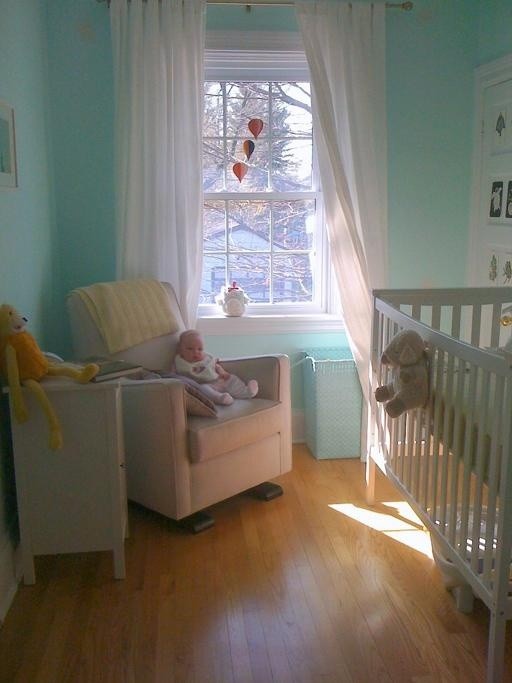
[145,368,218,418]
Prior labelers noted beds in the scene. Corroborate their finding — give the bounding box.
[366,287,512,683]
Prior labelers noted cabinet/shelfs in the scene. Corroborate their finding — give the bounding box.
[3,361,128,585]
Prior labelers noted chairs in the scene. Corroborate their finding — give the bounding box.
[68,281,293,534]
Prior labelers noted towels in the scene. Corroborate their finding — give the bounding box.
[67,278,180,354]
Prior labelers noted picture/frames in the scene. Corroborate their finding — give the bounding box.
[0,100,19,188]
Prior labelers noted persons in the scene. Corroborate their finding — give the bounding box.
[171,330,259,406]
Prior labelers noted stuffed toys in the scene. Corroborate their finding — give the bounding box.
[0,304,99,449]
[375,329,428,418]
